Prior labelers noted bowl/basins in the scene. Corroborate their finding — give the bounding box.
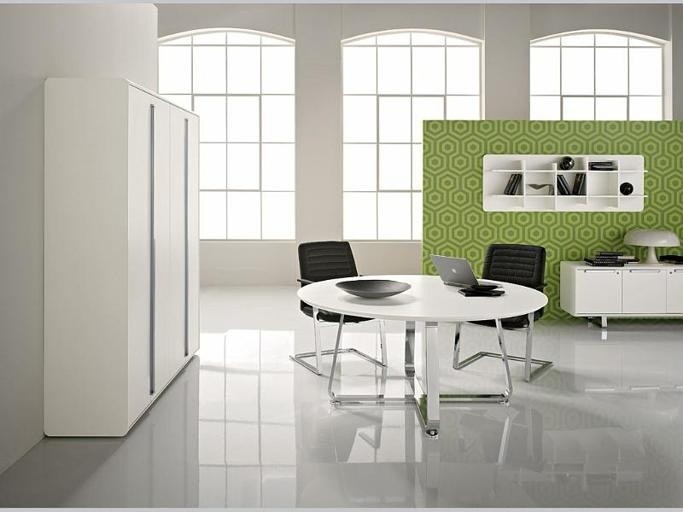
[335,279,412,300]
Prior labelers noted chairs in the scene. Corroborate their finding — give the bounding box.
[450,243,554,384]
[289,240,390,377]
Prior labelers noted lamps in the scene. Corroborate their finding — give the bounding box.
[622,227,681,262]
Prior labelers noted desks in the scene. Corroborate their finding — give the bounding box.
[297,273,549,438]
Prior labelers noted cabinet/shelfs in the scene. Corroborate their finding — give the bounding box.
[480,151,648,214]
[43,74,202,440]
[559,256,683,331]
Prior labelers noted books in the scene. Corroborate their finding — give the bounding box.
[459,289,505,296]
[557,173,586,195]
[584,251,640,267]
[504,173,522,195]
[589,161,617,170]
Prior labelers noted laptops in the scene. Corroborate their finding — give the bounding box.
[430,254,502,289]
